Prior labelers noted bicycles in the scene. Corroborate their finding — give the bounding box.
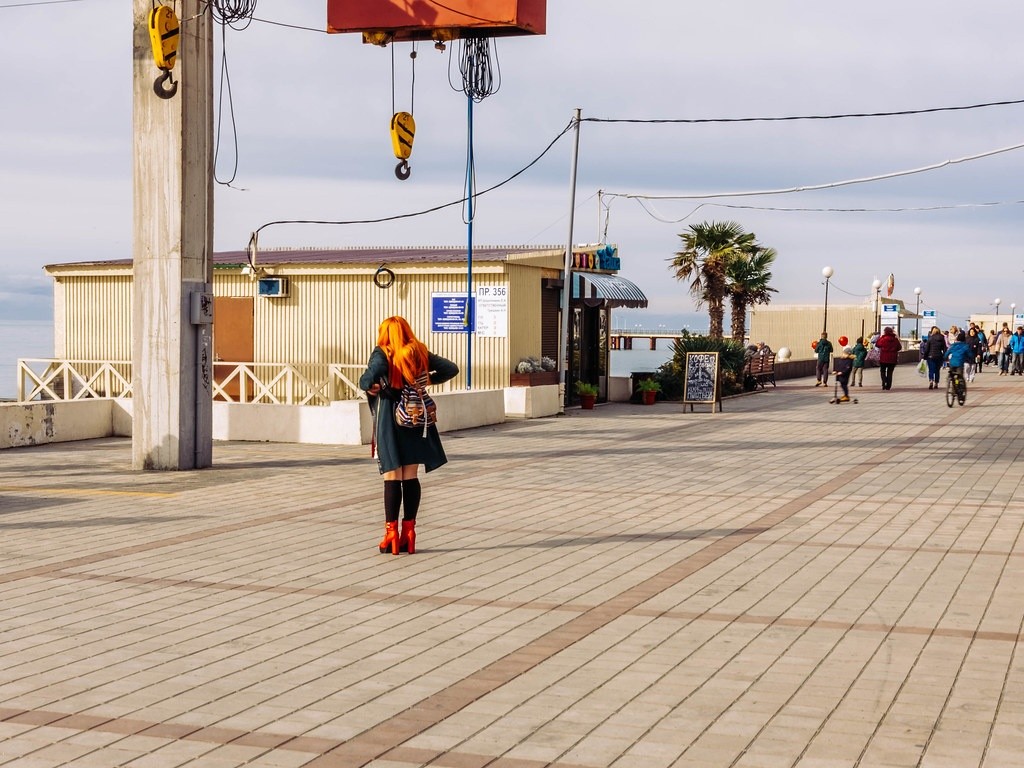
[945,360,971,408]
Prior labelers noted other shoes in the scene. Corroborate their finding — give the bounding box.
[859,383,862,387]
[851,382,854,386]
[886,386,890,390]
[929,383,933,389]
[934,383,938,389]
[815,381,822,387]
[966,369,982,382]
[999,369,1023,376]
[883,386,886,390]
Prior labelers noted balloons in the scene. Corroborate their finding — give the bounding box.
[812,341,817,349]
[839,336,848,346]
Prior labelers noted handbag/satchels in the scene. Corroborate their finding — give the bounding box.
[914,358,928,378]
[1003,347,1012,354]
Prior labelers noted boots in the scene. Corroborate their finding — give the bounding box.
[379,521,400,555]
[398,518,416,554]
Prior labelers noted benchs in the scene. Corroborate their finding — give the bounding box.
[742,352,777,389]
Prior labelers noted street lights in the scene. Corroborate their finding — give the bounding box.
[914,286,921,340]
[995,298,1001,334]
[1010,303,1016,333]
[873,279,881,332]
[822,266,834,333]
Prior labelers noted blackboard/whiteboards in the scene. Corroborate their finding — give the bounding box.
[683,351,722,402]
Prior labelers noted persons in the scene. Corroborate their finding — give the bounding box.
[851,337,867,387]
[871,328,902,390]
[832,347,856,401]
[816,333,833,387]
[920,322,1024,396]
[745,341,770,369]
[360,317,459,555]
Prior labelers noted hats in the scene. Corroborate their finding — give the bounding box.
[856,337,863,344]
[944,331,949,334]
[990,330,994,333]
[747,345,757,352]
[957,331,966,341]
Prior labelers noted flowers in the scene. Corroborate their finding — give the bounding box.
[517,356,556,373]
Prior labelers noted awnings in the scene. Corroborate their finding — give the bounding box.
[570,271,648,308]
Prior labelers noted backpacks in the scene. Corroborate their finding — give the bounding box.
[378,368,438,428]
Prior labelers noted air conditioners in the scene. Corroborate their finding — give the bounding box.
[256,276,291,298]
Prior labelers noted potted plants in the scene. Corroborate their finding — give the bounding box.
[575,380,600,410]
[635,378,663,405]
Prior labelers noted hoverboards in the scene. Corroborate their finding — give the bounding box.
[829,373,858,404]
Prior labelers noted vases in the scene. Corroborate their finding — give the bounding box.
[510,370,560,387]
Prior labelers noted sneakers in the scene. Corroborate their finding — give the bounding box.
[838,395,850,401]
